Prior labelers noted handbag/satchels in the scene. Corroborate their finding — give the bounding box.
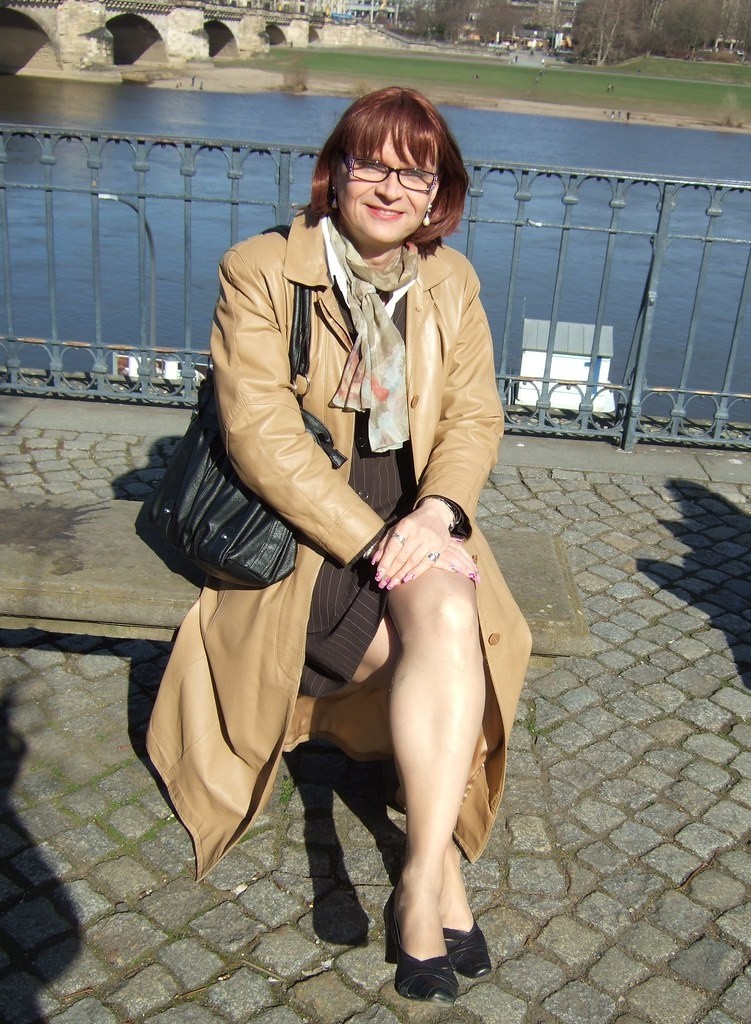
[149,224,348,592]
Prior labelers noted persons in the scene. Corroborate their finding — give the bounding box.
[727,112,732,124]
[638,69,641,75]
[471,46,554,83]
[606,82,615,93]
[145,87,533,1006]
[610,109,631,121]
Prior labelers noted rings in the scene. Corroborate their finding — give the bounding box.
[392,534,406,544]
[426,552,440,565]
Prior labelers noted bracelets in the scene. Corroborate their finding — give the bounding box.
[420,495,460,532]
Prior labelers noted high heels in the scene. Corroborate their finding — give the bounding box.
[383,887,460,1007]
[440,916,495,981]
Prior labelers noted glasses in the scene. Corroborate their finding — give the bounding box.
[341,148,441,192]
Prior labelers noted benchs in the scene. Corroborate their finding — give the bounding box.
[0,494,593,660]
